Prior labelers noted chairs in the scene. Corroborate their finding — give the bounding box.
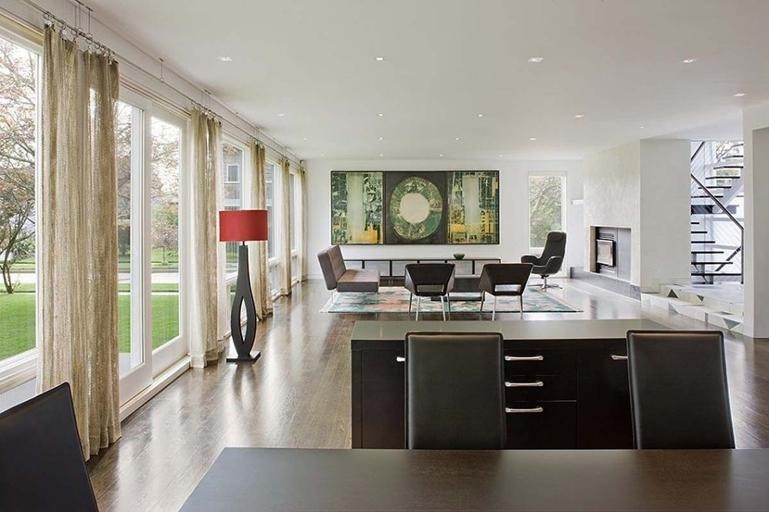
[1,382,102,512]
[627,332,735,449]
[403,331,506,451]
[403,263,455,321]
[479,263,534,320]
[522,231,567,291]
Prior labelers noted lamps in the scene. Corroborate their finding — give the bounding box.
[218,208,268,363]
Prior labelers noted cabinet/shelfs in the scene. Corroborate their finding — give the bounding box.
[580,338,636,449]
[349,340,580,449]
[343,258,502,285]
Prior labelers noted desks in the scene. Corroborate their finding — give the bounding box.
[173,446,768,512]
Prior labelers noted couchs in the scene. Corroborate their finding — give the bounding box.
[316,243,381,318]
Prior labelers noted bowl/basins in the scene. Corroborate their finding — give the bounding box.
[454,254,465,259]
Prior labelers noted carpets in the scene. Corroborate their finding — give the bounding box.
[318,283,584,314]
